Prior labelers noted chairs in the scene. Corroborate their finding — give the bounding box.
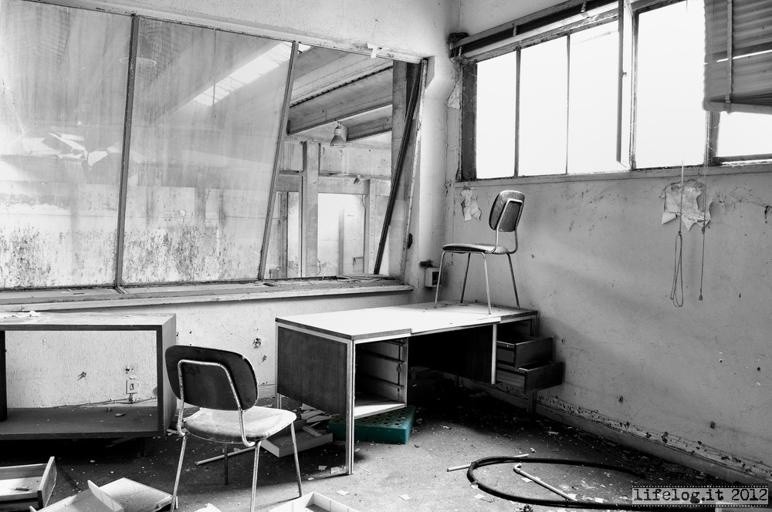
[165,344,303,512]
[433,190,526,315]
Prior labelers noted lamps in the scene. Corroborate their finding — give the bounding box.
[330,124,347,148]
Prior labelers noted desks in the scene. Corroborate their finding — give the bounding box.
[275,302,565,476]
[0,311,177,463]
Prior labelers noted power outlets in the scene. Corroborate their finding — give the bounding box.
[126,380,141,395]
